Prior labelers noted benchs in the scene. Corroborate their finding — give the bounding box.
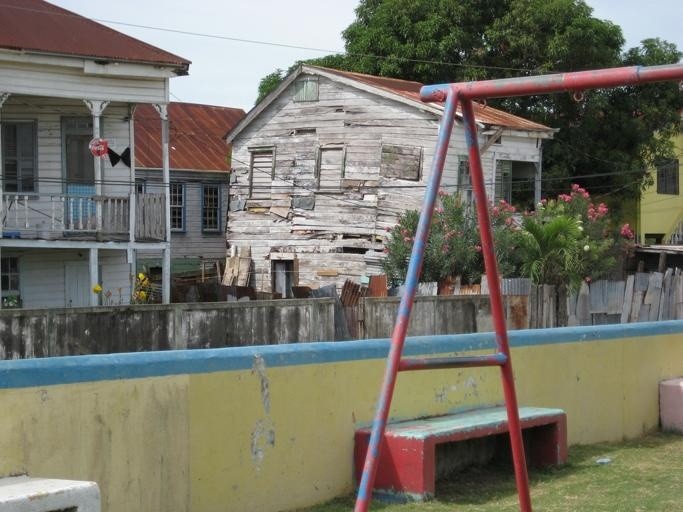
[355,406,568,503]
[659,376,683,436]
[0,474,102,512]
